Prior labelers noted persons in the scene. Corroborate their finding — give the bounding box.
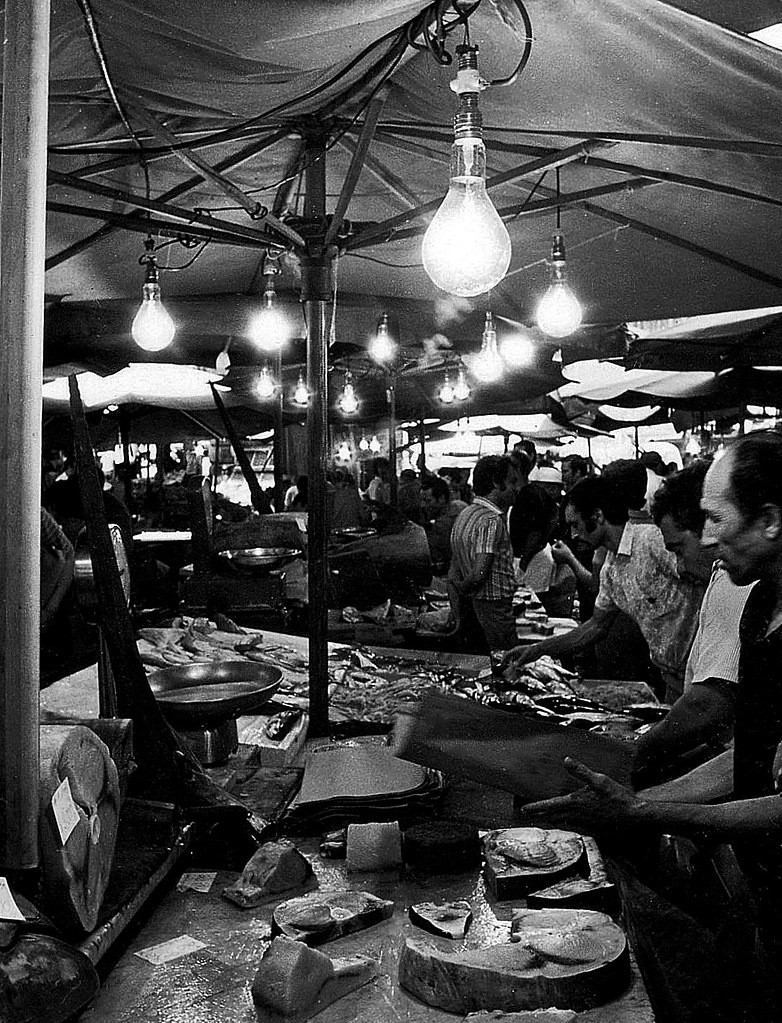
[32,415,782,1021]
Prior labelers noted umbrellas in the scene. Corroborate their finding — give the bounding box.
[1,2,780,743]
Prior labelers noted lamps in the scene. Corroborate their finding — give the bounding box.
[470,311,502,382]
[250,283,288,351]
[440,373,454,402]
[294,375,309,403]
[256,361,274,396]
[369,312,396,361]
[340,371,357,412]
[421,44,512,298]
[131,269,175,351]
[454,372,469,399]
[537,234,582,338]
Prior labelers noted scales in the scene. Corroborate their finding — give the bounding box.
[74,523,284,767]
[180,475,304,606]
